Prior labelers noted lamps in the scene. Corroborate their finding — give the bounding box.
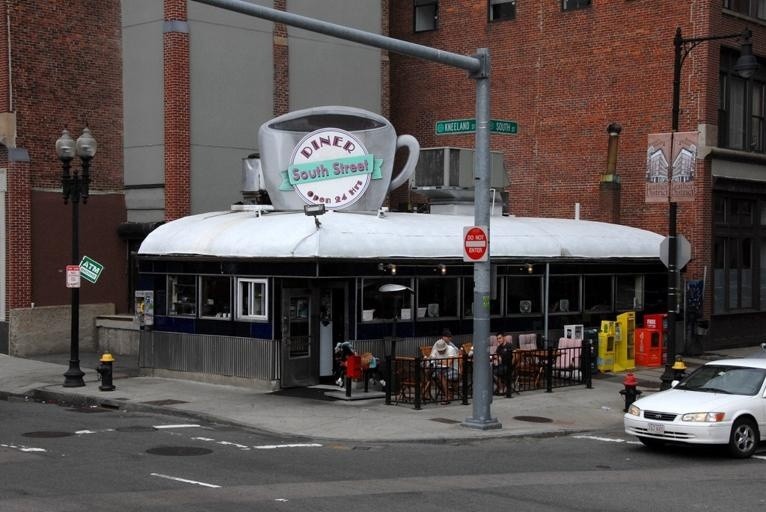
[304,204,325,229]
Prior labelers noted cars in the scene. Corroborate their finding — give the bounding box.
[624,358,766,459]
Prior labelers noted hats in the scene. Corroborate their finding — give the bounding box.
[436,340,447,351]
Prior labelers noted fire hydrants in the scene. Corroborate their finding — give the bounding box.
[619,370,642,413]
[672,358,687,380]
[96,353,115,391]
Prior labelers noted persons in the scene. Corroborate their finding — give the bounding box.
[441,330,459,350]
[427,338,460,405]
[490,330,518,395]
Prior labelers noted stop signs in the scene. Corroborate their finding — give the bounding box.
[463,225,489,262]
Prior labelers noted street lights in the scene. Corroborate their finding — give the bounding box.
[660,26,758,391]
[56,127,97,387]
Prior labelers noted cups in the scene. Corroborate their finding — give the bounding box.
[258,105,421,218]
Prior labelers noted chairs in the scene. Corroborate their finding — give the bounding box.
[394,334,581,409]
